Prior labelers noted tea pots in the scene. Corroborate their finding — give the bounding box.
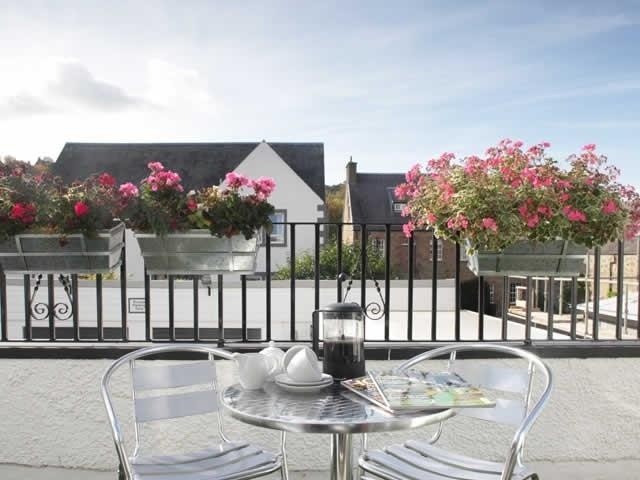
[310,301,367,383]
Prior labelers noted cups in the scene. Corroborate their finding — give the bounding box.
[235,342,284,389]
[280,344,322,381]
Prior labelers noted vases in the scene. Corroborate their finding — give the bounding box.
[14,220,261,279]
[465,234,588,278]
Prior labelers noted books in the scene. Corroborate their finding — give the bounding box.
[368,368,498,411]
[340,389,397,418]
[338,374,427,416]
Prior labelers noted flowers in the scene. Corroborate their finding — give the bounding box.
[393,137,640,257]
[0,155,277,239]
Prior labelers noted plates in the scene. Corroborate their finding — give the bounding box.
[273,373,336,395]
